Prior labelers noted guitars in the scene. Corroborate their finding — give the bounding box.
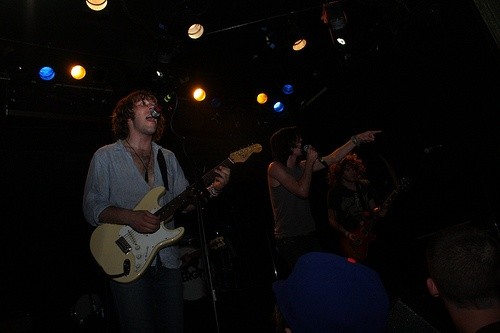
[339,175,412,265]
[89,142,263,286]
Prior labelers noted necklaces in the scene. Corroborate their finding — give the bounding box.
[124,139,150,184]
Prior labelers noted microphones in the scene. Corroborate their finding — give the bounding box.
[304,145,328,167]
[151,92,174,118]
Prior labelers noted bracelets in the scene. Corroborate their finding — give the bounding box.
[351,135,360,144]
[207,183,220,197]
[345,231,349,236]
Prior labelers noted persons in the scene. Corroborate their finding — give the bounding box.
[179,247,206,333]
[424,216,500,333]
[327,152,385,255]
[83,90,231,333]
[268,126,381,333]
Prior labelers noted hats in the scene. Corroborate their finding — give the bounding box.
[272,251,391,333]
